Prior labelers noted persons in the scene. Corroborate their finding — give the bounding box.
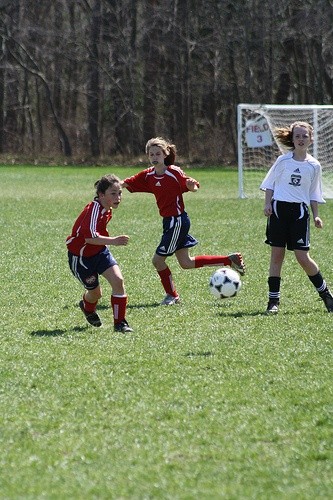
[260,121,333,314]
[120,138,245,305]
[65,175,134,332]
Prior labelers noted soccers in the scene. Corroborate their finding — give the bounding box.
[209,267,241,298]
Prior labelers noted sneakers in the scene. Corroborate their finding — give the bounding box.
[266,300,279,314]
[161,293,181,307]
[324,296,333,312]
[79,301,101,327]
[114,319,134,334]
[228,253,245,276]
